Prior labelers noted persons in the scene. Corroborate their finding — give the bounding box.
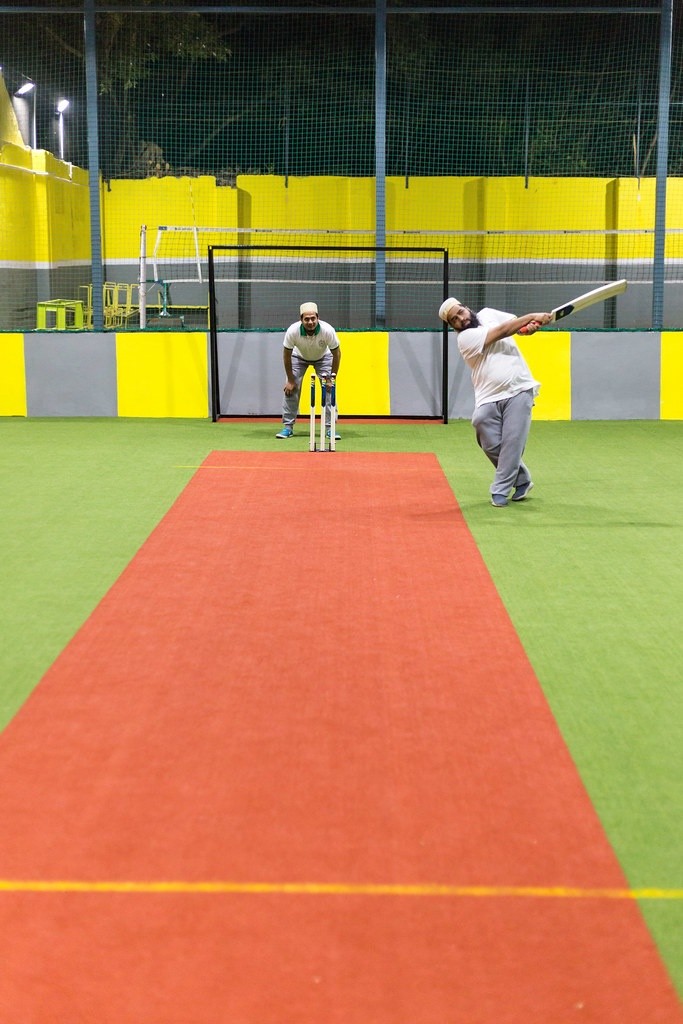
[275,302,341,439]
[439,297,554,507]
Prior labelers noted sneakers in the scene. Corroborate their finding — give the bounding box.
[276,428,293,439]
[326,431,341,439]
[491,494,507,506]
[512,482,534,500]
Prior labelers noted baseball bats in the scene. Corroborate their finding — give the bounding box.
[310,373,316,451]
[517,279,628,334]
[330,373,336,451]
[320,373,326,452]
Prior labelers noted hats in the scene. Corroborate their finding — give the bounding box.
[300,302,318,314]
[439,297,461,322]
